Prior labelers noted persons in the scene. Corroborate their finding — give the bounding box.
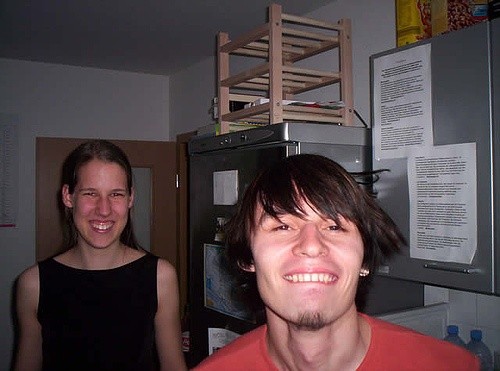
[9,140,188,371]
[186,153,481,371]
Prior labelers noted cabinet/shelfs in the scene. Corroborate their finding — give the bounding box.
[370,19,493,298]
[217,4,355,135]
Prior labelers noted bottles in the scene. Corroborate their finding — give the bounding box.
[443,325,465,348]
[466,330,494,371]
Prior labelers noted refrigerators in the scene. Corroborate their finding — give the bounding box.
[187,122,372,369]
